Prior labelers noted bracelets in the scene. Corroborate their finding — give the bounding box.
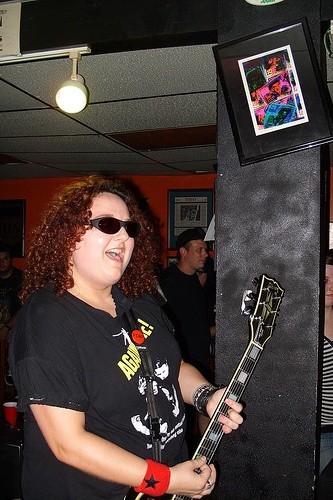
[194,385,220,418]
[5,324,11,329]
[134,459,170,497]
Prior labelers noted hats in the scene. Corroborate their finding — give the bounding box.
[175,228,203,249]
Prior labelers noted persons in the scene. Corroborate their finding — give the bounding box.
[161,228,217,387]
[319,256,333,474]
[0,245,22,385]
[7,177,243,500]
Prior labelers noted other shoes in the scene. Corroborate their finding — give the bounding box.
[4,362,15,385]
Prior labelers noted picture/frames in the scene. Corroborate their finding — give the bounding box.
[211,16,333,166]
[167,188,215,250]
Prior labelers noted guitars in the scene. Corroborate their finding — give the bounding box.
[121,272,287,500]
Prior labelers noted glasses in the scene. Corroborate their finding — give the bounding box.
[80,216,141,238]
[0,257,11,263]
[196,271,208,276]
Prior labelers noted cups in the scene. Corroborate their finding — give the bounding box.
[3,402,17,427]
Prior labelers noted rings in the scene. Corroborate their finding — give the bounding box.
[207,480,213,488]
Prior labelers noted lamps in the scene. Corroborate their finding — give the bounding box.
[56,53,89,113]
